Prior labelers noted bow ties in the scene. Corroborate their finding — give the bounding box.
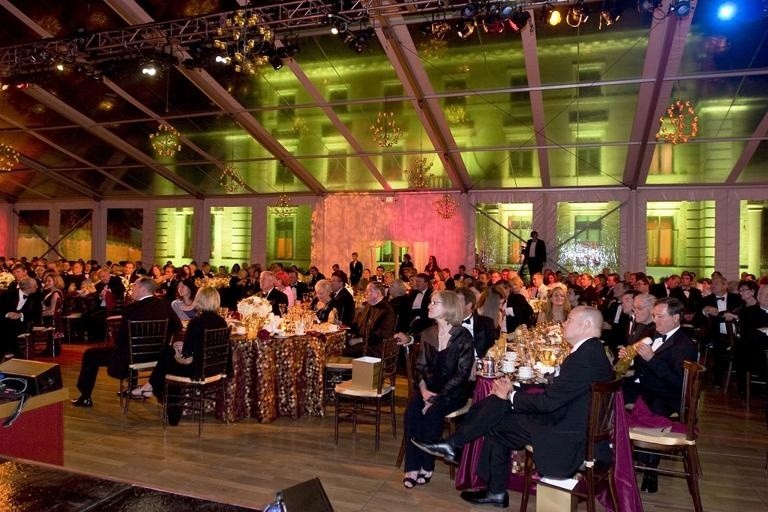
[461,319,470,324]
[23,295,29,299]
[683,288,690,291]
[416,291,423,295]
[378,278,382,280]
[262,293,268,298]
[629,316,633,322]
[655,333,668,342]
[716,297,725,301]
[616,302,622,307]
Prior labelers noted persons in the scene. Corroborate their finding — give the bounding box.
[411,304,614,508]
[615,295,698,495]
[520,231,547,283]
[1,249,767,429]
[400,289,477,490]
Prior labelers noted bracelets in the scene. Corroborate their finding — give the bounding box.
[505,387,514,400]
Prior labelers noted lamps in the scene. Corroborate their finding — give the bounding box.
[654,75,698,148]
[147,74,183,158]
[1,0,694,96]
[401,122,435,189]
[215,124,246,195]
[211,10,268,76]
[0,144,20,171]
[370,52,402,151]
[429,174,461,220]
[272,165,295,217]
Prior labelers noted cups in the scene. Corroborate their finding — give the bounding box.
[219,300,340,340]
[482,320,573,381]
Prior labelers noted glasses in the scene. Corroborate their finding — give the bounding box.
[431,300,444,305]
[739,288,752,292]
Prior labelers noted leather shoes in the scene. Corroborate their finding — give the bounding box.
[411,436,460,466]
[71,398,93,408]
[117,389,131,397]
[460,489,509,508]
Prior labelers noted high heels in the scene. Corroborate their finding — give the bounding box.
[126,386,153,404]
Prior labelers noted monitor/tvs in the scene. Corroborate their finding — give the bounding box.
[262,478,334,512]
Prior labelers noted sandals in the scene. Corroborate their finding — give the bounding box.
[404,468,433,488]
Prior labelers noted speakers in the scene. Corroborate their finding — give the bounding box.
[0,358,62,395]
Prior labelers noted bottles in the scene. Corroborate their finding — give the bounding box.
[634,337,652,351]
[612,345,637,376]
[70,282,76,291]
